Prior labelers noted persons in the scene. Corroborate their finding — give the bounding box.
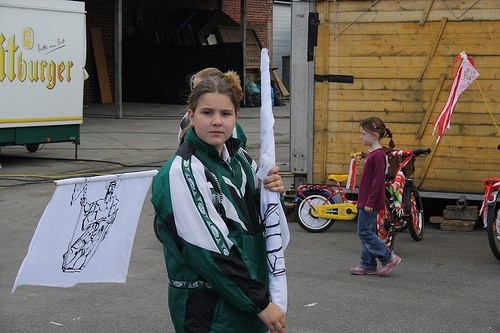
[350,117,401,277]
[151,68,288,333]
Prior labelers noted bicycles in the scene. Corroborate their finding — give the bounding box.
[294,150,369,233]
[480,140,500,261]
[375,148,432,250]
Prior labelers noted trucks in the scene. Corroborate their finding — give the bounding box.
[0,1,90,151]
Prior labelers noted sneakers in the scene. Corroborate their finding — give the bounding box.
[349,266,377,275]
[377,251,402,276]
